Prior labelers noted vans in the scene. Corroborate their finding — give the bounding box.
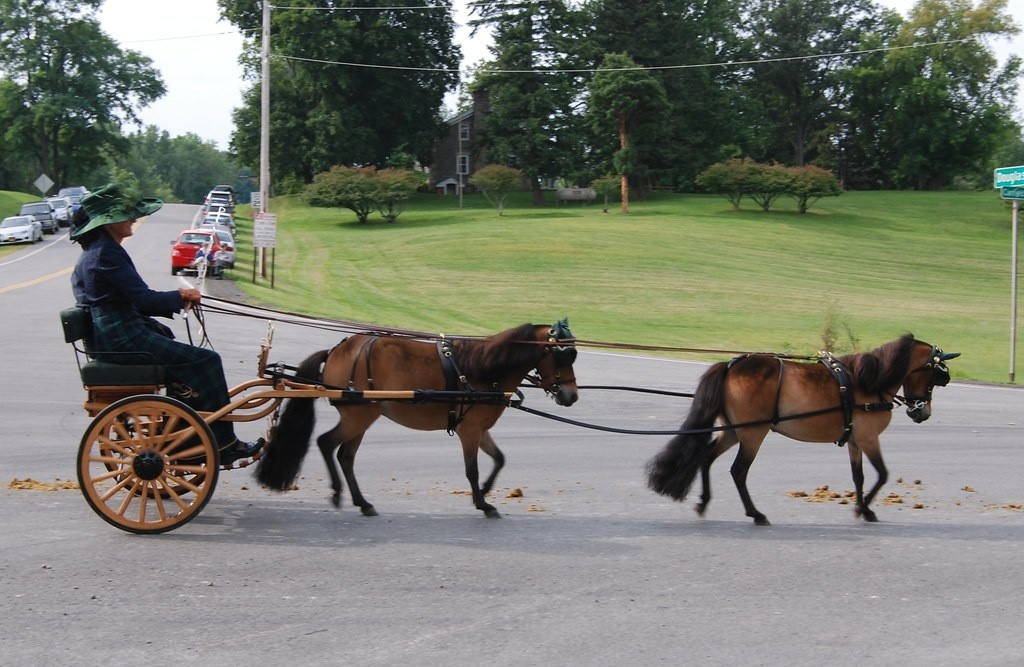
[57,185,91,214]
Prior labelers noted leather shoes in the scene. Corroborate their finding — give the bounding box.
[219,437,266,466]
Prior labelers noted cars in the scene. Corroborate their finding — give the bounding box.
[169,182,238,279]
[14,201,59,234]
[45,195,74,227]
[0,215,43,245]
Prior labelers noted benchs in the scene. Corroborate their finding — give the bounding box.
[60,307,166,391]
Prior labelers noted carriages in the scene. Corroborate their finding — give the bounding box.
[59,291,961,535]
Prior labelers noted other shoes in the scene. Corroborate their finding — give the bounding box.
[215,277,223,280]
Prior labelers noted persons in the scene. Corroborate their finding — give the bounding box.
[70,182,265,466]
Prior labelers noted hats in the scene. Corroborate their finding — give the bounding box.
[69,181,163,239]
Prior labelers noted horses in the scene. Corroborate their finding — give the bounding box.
[252,315,579,518]
[640,332,961,528]
